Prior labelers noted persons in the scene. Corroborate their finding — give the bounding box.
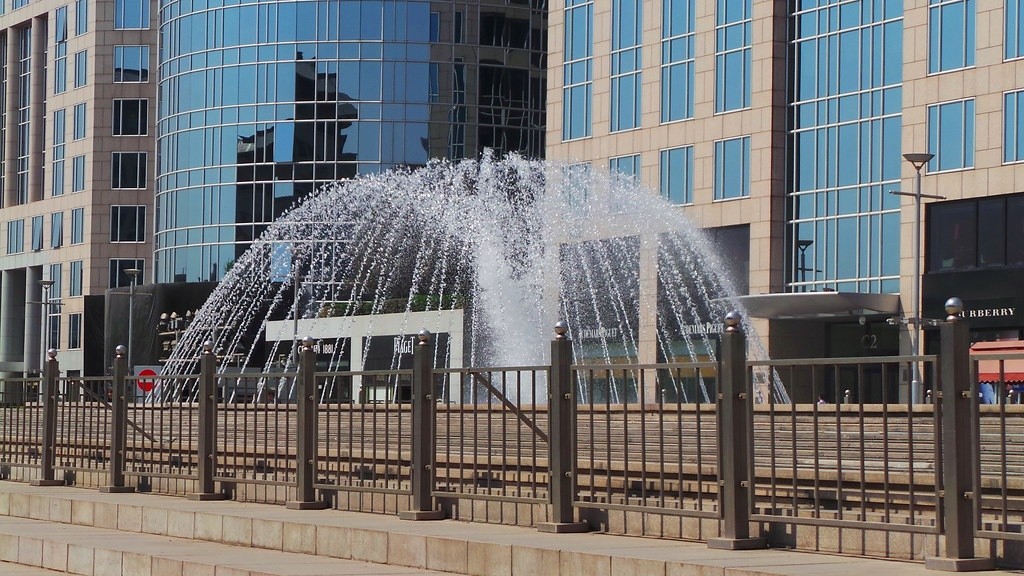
[981,382,995,405]
[259,389,281,404]
[817,393,830,404]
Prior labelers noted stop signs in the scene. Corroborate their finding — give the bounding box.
[137,369,159,393]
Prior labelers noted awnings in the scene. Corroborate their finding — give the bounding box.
[970,341,1024,382]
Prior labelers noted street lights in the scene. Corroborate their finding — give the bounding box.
[37,279,58,377]
[124,268,143,388]
[902,152,936,404]
